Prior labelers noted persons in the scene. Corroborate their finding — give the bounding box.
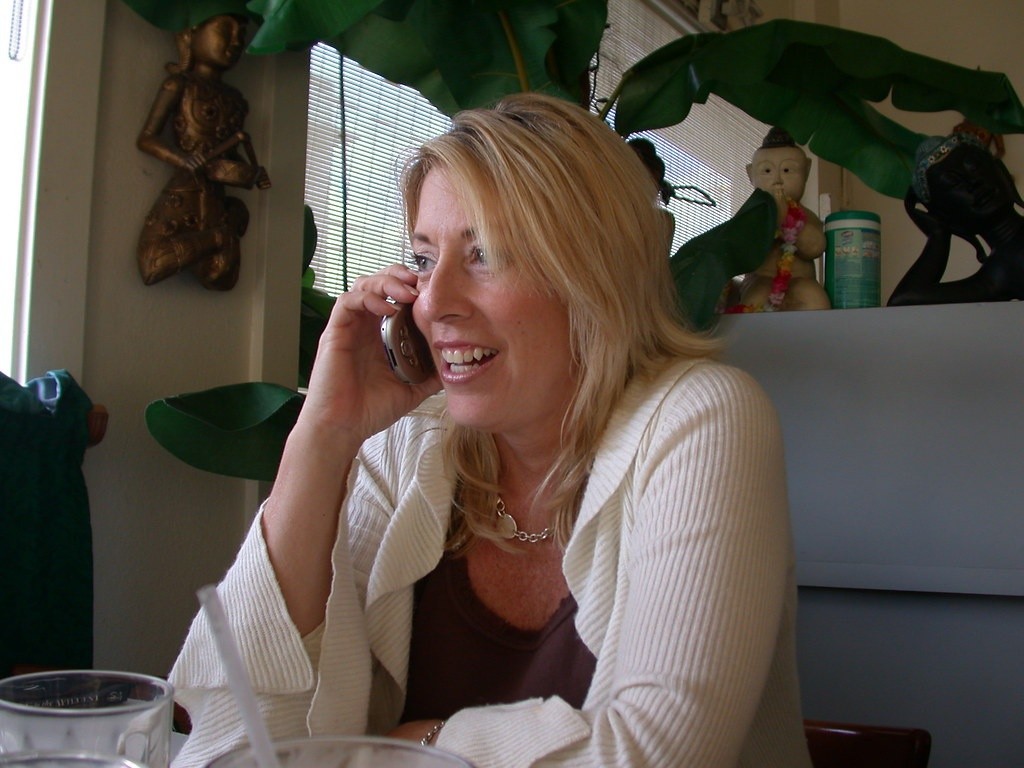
[163,89,812,768]
[735,125,832,312]
[134,13,274,294]
[885,115,1024,307]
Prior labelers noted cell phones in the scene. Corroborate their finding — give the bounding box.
[380,300,436,383]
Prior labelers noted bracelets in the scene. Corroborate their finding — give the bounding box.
[417,715,450,748]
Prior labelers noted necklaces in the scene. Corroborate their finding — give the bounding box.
[495,496,559,543]
[719,196,808,314]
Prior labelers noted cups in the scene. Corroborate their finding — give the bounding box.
[0,669,175,768]
[201,735,477,768]
[823,208,881,308]
[0,750,151,768]
